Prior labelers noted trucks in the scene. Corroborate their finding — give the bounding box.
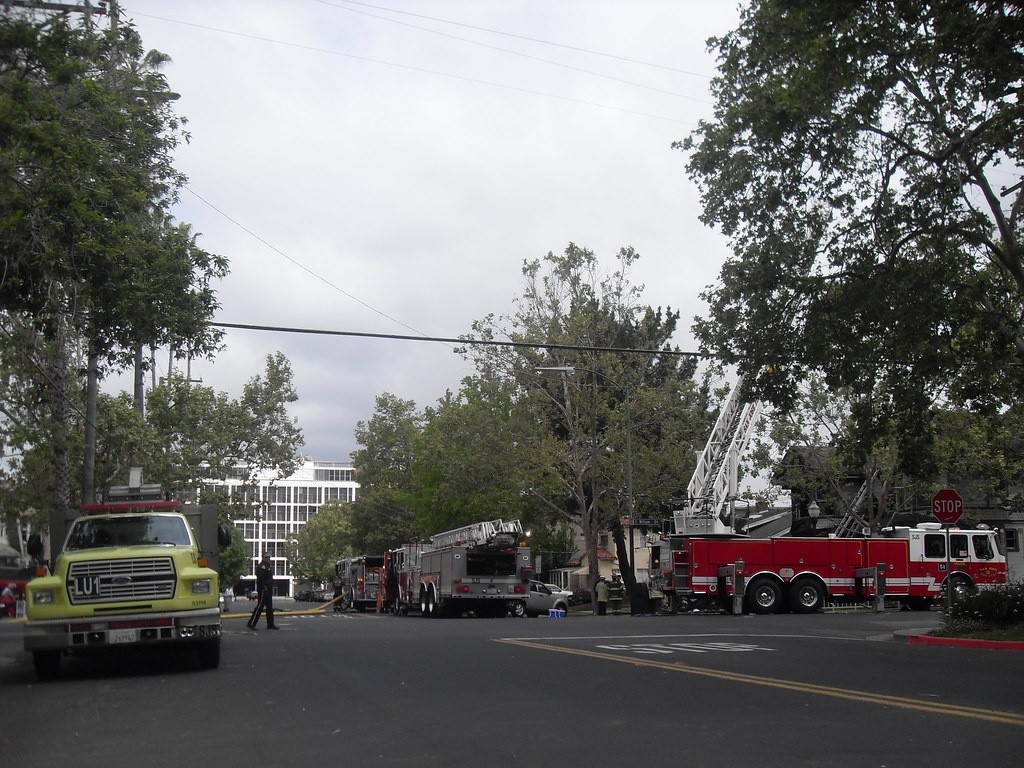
[23,463,230,680]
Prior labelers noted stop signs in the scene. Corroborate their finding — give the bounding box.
[931,488,964,524]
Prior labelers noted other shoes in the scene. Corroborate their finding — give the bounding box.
[246,622,256,633]
[267,624,279,629]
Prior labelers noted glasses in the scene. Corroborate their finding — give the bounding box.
[264,556,270,558]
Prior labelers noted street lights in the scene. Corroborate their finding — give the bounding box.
[535,366,635,583]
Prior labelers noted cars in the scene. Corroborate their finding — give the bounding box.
[524,580,570,620]
[536,583,575,606]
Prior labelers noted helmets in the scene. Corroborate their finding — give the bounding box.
[611,573,616,577]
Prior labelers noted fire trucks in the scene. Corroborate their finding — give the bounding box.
[645,252,1013,616]
[382,519,533,620]
[332,554,387,614]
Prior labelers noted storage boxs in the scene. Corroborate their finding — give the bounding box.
[548,608,565,619]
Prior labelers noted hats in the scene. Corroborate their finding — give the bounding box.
[600,576,606,579]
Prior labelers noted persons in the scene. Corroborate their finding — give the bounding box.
[295,589,320,603]
[225,586,233,595]
[2,582,18,618]
[245,552,279,631]
[595,575,610,616]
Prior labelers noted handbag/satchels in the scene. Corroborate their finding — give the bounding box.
[4,596,14,604]
